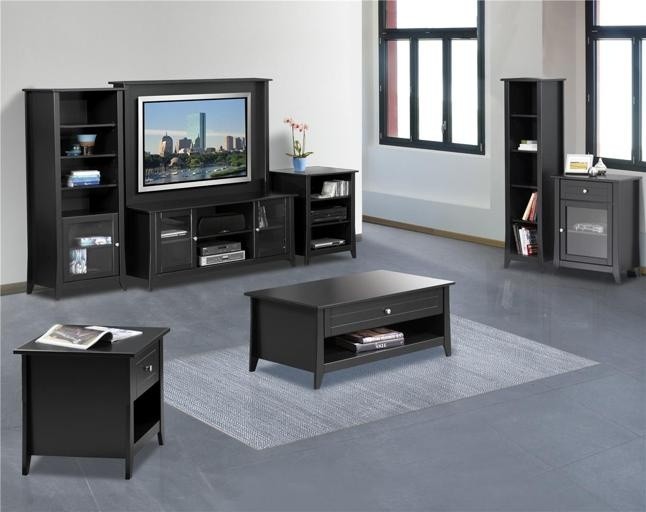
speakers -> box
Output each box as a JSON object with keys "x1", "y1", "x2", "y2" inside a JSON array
[{"x1": 197, "y1": 212, "x2": 246, "y2": 236}]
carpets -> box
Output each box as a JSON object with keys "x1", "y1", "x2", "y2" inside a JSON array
[{"x1": 156, "y1": 311, "x2": 602, "y2": 454}]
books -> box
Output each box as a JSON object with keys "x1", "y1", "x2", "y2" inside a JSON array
[
  {"x1": 35, "y1": 322, "x2": 144, "y2": 350},
  {"x1": 311, "y1": 178, "x2": 349, "y2": 199},
  {"x1": 330, "y1": 328, "x2": 405, "y2": 354},
  {"x1": 257, "y1": 201, "x2": 268, "y2": 230},
  {"x1": 61, "y1": 169, "x2": 101, "y2": 189},
  {"x1": 517, "y1": 139, "x2": 537, "y2": 152},
  {"x1": 513, "y1": 192, "x2": 539, "y2": 256},
  {"x1": 162, "y1": 228, "x2": 188, "y2": 239}
]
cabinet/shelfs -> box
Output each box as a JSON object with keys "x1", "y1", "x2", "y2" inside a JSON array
[
  {"x1": 498, "y1": 76, "x2": 566, "y2": 274},
  {"x1": 123, "y1": 190, "x2": 302, "y2": 292},
  {"x1": 548, "y1": 168, "x2": 643, "y2": 285},
  {"x1": 20, "y1": 85, "x2": 130, "y2": 301},
  {"x1": 10, "y1": 321, "x2": 167, "y2": 484},
  {"x1": 270, "y1": 163, "x2": 361, "y2": 267}
]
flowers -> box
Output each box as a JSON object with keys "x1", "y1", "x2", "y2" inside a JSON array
[{"x1": 281, "y1": 115, "x2": 317, "y2": 159}]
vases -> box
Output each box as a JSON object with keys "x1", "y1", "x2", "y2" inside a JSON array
[{"x1": 289, "y1": 157, "x2": 310, "y2": 172}]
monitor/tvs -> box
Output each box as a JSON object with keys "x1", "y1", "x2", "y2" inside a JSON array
[{"x1": 136, "y1": 92, "x2": 251, "y2": 194}]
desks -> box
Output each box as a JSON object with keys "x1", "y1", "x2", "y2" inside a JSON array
[{"x1": 240, "y1": 270, "x2": 458, "y2": 392}]
